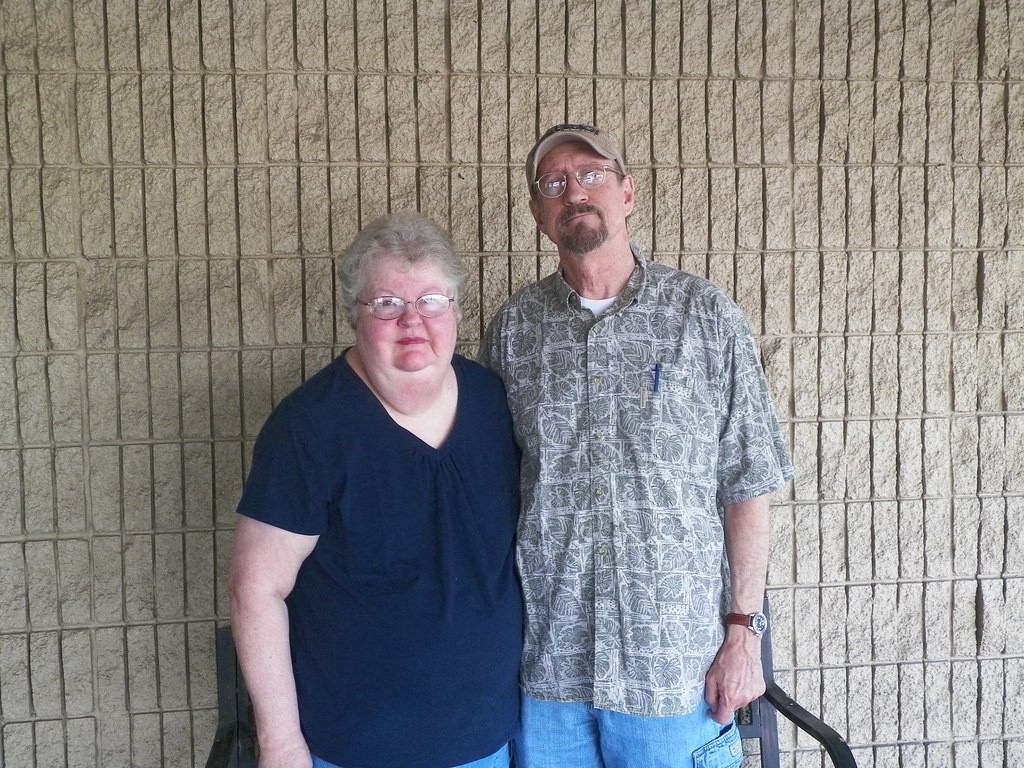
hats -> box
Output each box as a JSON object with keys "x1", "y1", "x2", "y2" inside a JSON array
[{"x1": 525, "y1": 123, "x2": 627, "y2": 197}]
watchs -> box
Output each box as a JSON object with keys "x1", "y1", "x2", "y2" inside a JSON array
[{"x1": 723, "y1": 611, "x2": 768, "y2": 637}]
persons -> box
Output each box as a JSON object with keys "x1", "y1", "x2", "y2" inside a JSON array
[
  {"x1": 473, "y1": 123, "x2": 799, "y2": 768},
  {"x1": 231, "y1": 210, "x2": 527, "y2": 768}
]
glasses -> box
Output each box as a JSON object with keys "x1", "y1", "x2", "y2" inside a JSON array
[
  {"x1": 534, "y1": 163, "x2": 626, "y2": 199},
  {"x1": 354, "y1": 293, "x2": 455, "y2": 320}
]
